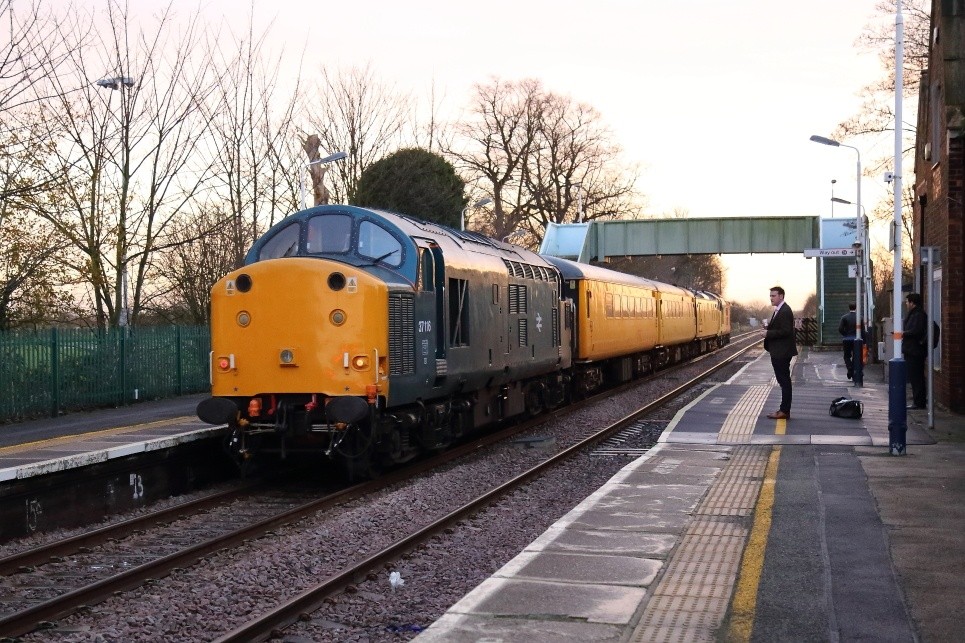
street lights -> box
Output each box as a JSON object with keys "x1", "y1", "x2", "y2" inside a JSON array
[
  {"x1": 299, "y1": 152, "x2": 348, "y2": 210},
  {"x1": 831, "y1": 197, "x2": 869, "y2": 365},
  {"x1": 843, "y1": 222, "x2": 873, "y2": 333},
  {"x1": 96, "y1": 76, "x2": 134, "y2": 405},
  {"x1": 808, "y1": 134, "x2": 864, "y2": 388}
]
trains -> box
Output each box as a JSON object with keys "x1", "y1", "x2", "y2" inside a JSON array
[{"x1": 195, "y1": 205, "x2": 732, "y2": 486}]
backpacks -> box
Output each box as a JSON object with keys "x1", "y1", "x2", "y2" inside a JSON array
[{"x1": 915, "y1": 315, "x2": 941, "y2": 350}]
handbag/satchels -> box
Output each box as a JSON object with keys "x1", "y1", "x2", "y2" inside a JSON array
[{"x1": 829, "y1": 397, "x2": 865, "y2": 419}]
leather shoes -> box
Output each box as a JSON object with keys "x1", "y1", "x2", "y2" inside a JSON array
[
  {"x1": 766, "y1": 409, "x2": 790, "y2": 419},
  {"x1": 906, "y1": 404, "x2": 926, "y2": 409},
  {"x1": 847, "y1": 367, "x2": 854, "y2": 379}
]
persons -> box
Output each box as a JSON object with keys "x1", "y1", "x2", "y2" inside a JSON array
[
  {"x1": 760, "y1": 287, "x2": 798, "y2": 418},
  {"x1": 839, "y1": 303, "x2": 866, "y2": 379},
  {"x1": 903, "y1": 292, "x2": 928, "y2": 409}
]
